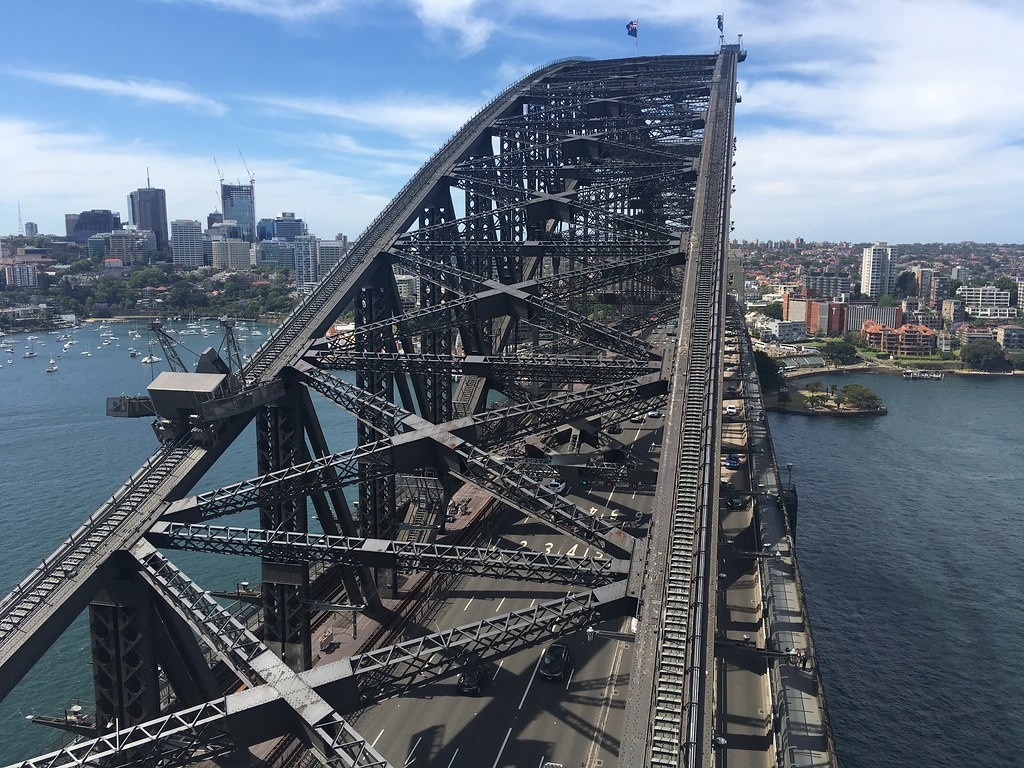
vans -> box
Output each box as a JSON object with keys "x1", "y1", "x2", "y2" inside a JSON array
[
  {"x1": 648, "y1": 404, "x2": 659, "y2": 417},
  {"x1": 724, "y1": 363, "x2": 735, "y2": 372},
  {"x1": 724, "y1": 450, "x2": 743, "y2": 470}
]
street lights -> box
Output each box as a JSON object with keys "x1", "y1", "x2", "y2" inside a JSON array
[{"x1": 787, "y1": 463, "x2": 795, "y2": 491}]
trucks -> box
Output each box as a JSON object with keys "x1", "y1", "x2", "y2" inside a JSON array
[{"x1": 665, "y1": 325, "x2": 676, "y2": 335}]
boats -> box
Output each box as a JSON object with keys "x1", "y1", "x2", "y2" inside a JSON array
[
  {"x1": 902, "y1": 370, "x2": 944, "y2": 380},
  {"x1": 0, "y1": 309, "x2": 273, "y2": 372}
]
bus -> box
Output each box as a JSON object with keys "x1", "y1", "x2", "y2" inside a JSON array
[{"x1": 785, "y1": 366, "x2": 798, "y2": 370}]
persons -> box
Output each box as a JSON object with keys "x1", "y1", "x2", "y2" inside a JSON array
[
  {"x1": 801, "y1": 648, "x2": 808, "y2": 671},
  {"x1": 784, "y1": 647, "x2": 790, "y2": 670},
  {"x1": 794, "y1": 648, "x2": 800, "y2": 670},
  {"x1": 777, "y1": 495, "x2": 780, "y2": 509}
]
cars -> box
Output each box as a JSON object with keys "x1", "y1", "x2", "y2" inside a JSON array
[
  {"x1": 652, "y1": 329, "x2": 658, "y2": 334},
  {"x1": 658, "y1": 325, "x2": 662, "y2": 329},
  {"x1": 608, "y1": 422, "x2": 623, "y2": 435},
  {"x1": 725, "y1": 496, "x2": 747, "y2": 510},
  {"x1": 629, "y1": 410, "x2": 642, "y2": 422},
  {"x1": 535, "y1": 640, "x2": 570, "y2": 680},
  {"x1": 726, "y1": 343, "x2": 735, "y2": 352}
]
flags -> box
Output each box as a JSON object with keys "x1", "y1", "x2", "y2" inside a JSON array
[
  {"x1": 626, "y1": 21, "x2": 637, "y2": 37},
  {"x1": 717, "y1": 15, "x2": 723, "y2": 32}
]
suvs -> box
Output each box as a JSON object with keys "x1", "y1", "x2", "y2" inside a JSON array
[
  {"x1": 726, "y1": 404, "x2": 737, "y2": 416},
  {"x1": 548, "y1": 475, "x2": 567, "y2": 494}
]
